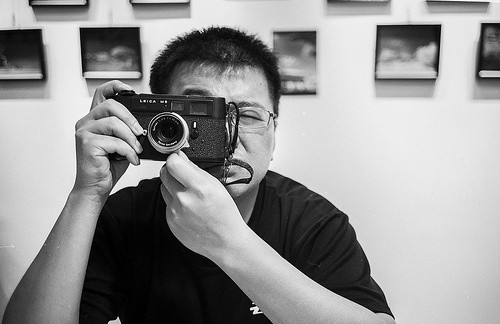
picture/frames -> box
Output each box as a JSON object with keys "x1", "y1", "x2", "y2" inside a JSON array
[
  {"x1": 475, "y1": 22, "x2": 500, "y2": 80},
  {"x1": 0, "y1": 29, "x2": 46, "y2": 82},
  {"x1": 80, "y1": 26, "x2": 143, "y2": 81},
  {"x1": 272, "y1": 31, "x2": 317, "y2": 96},
  {"x1": 374, "y1": 24, "x2": 442, "y2": 81}
]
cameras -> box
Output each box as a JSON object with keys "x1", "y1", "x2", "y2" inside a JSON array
[{"x1": 106, "y1": 90, "x2": 230, "y2": 165}]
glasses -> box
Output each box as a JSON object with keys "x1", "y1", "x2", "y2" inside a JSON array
[{"x1": 232, "y1": 107, "x2": 277, "y2": 129}]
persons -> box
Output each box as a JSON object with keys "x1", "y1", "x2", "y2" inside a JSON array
[{"x1": 2, "y1": 24, "x2": 398, "y2": 324}]
